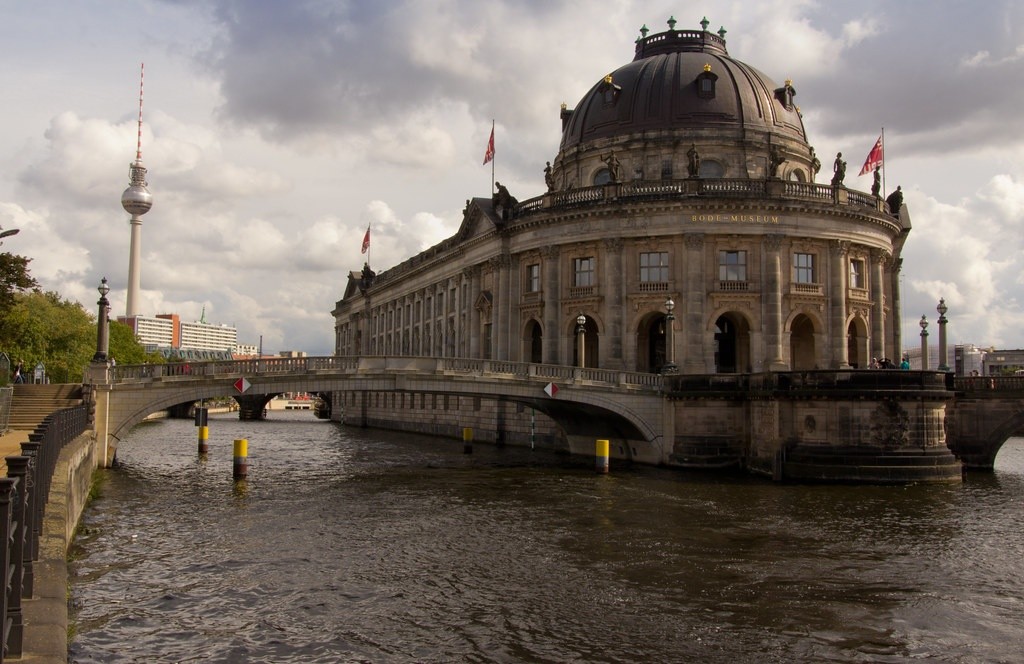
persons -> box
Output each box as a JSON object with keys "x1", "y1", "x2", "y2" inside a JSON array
[
  {"x1": 867, "y1": 357, "x2": 909, "y2": 370},
  {"x1": 686, "y1": 141, "x2": 701, "y2": 178},
  {"x1": 600, "y1": 148, "x2": 621, "y2": 183},
  {"x1": 544, "y1": 161, "x2": 556, "y2": 193},
  {"x1": 111, "y1": 357, "x2": 116, "y2": 367},
  {"x1": 12, "y1": 361, "x2": 24, "y2": 383},
  {"x1": 769, "y1": 144, "x2": 903, "y2": 213},
  {"x1": 494, "y1": 181, "x2": 511, "y2": 209},
  {"x1": 143, "y1": 360, "x2": 148, "y2": 374}
]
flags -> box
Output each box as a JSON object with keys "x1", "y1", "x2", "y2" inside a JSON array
[
  {"x1": 361, "y1": 227, "x2": 371, "y2": 254},
  {"x1": 857, "y1": 135, "x2": 883, "y2": 178},
  {"x1": 482, "y1": 127, "x2": 495, "y2": 165}
]
